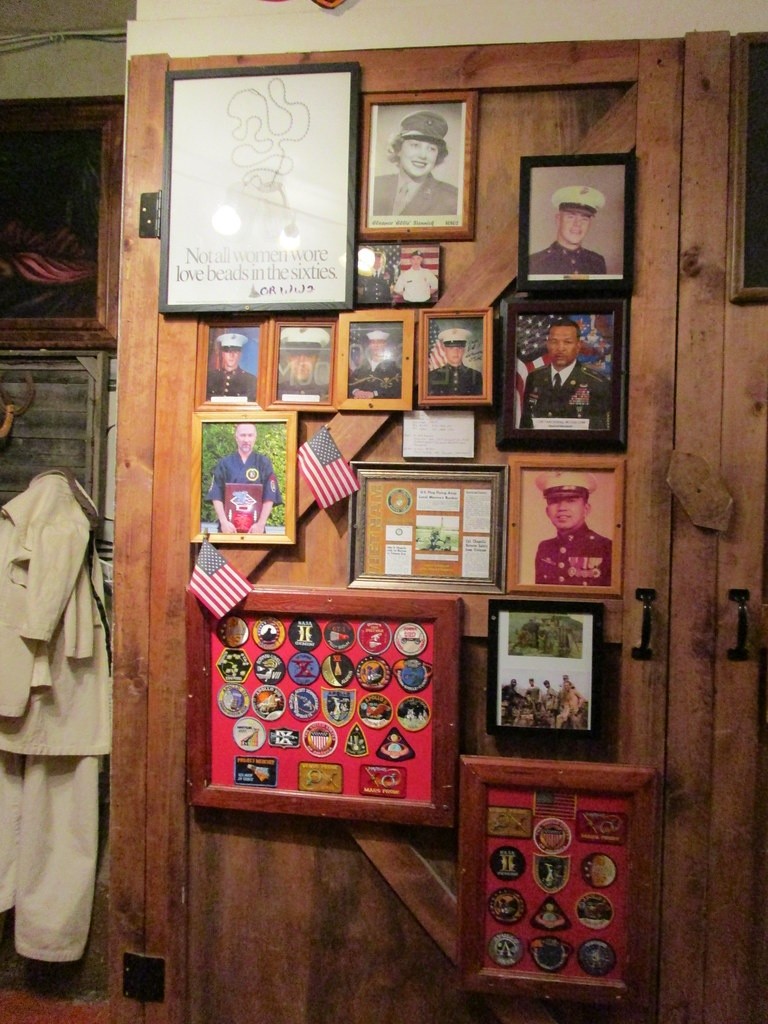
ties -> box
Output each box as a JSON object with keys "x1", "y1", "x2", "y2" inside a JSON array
[
  {"x1": 375, "y1": 271, "x2": 378, "y2": 278},
  {"x1": 553, "y1": 373, "x2": 562, "y2": 397}
]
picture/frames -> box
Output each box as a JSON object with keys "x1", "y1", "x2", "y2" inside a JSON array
[
  {"x1": 506, "y1": 453, "x2": 626, "y2": 595},
  {"x1": 158, "y1": 60, "x2": 361, "y2": 313},
  {"x1": 188, "y1": 592, "x2": 460, "y2": 828},
  {"x1": 730, "y1": 29, "x2": 768, "y2": 309},
  {"x1": 266, "y1": 316, "x2": 339, "y2": 413},
  {"x1": 518, "y1": 151, "x2": 637, "y2": 292},
  {"x1": 356, "y1": 92, "x2": 478, "y2": 242},
  {"x1": 189, "y1": 412, "x2": 297, "y2": 546},
  {"x1": 485, "y1": 601, "x2": 605, "y2": 737},
  {"x1": 354, "y1": 469, "x2": 501, "y2": 586},
  {"x1": 195, "y1": 318, "x2": 268, "y2": 412},
  {"x1": 455, "y1": 760, "x2": 659, "y2": 998},
  {"x1": 0, "y1": 94, "x2": 122, "y2": 349},
  {"x1": 338, "y1": 310, "x2": 414, "y2": 412},
  {"x1": 497, "y1": 298, "x2": 632, "y2": 449},
  {"x1": 417, "y1": 307, "x2": 494, "y2": 405}
]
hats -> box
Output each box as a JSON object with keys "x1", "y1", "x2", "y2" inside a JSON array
[
  {"x1": 280, "y1": 326, "x2": 331, "y2": 355},
  {"x1": 399, "y1": 110, "x2": 448, "y2": 147},
  {"x1": 411, "y1": 249, "x2": 424, "y2": 257},
  {"x1": 217, "y1": 332, "x2": 248, "y2": 351},
  {"x1": 438, "y1": 328, "x2": 473, "y2": 347},
  {"x1": 536, "y1": 467, "x2": 598, "y2": 498},
  {"x1": 374, "y1": 247, "x2": 383, "y2": 256},
  {"x1": 551, "y1": 184, "x2": 606, "y2": 217},
  {"x1": 366, "y1": 329, "x2": 390, "y2": 341}
]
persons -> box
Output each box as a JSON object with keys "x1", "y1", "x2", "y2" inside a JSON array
[
  {"x1": 427, "y1": 328, "x2": 483, "y2": 396},
  {"x1": 394, "y1": 250, "x2": 439, "y2": 302},
  {"x1": 519, "y1": 319, "x2": 610, "y2": 430},
  {"x1": 533, "y1": 469, "x2": 611, "y2": 586},
  {"x1": 206, "y1": 334, "x2": 258, "y2": 403},
  {"x1": 373, "y1": 110, "x2": 457, "y2": 216},
  {"x1": 501, "y1": 674, "x2": 588, "y2": 729},
  {"x1": 348, "y1": 331, "x2": 400, "y2": 398},
  {"x1": 204, "y1": 422, "x2": 282, "y2": 534},
  {"x1": 277, "y1": 328, "x2": 330, "y2": 399},
  {"x1": 520, "y1": 615, "x2": 563, "y2": 659},
  {"x1": 527, "y1": 185, "x2": 607, "y2": 273},
  {"x1": 356, "y1": 248, "x2": 393, "y2": 302}
]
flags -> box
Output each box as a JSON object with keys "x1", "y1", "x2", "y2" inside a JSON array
[
  {"x1": 349, "y1": 322, "x2": 369, "y2": 376},
  {"x1": 517, "y1": 315, "x2": 562, "y2": 429},
  {"x1": 295, "y1": 426, "x2": 360, "y2": 510},
  {"x1": 428, "y1": 319, "x2": 447, "y2": 370},
  {"x1": 186, "y1": 538, "x2": 256, "y2": 621},
  {"x1": 357, "y1": 245, "x2": 439, "y2": 302}
]
